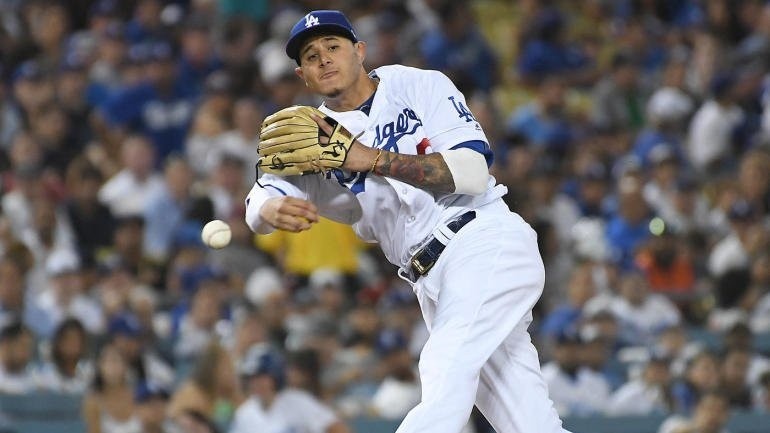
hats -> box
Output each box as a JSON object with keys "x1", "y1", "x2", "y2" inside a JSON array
[{"x1": 286, "y1": 10, "x2": 357, "y2": 60}]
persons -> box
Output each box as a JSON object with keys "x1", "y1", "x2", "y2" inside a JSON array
[
  {"x1": 244, "y1": 11, "x2": 572, "y2": 433},
  {"x1": 0, "y1": 0, "x2": 770, "y2": 433}
]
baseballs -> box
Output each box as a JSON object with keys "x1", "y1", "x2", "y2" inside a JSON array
[{"x1": 202, "y1": 220, "x2": 232, "y2": 250}]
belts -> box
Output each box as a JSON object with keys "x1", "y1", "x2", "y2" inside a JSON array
[{"x1": 411, "y1": 211, "x2": 475, "y2": 282}]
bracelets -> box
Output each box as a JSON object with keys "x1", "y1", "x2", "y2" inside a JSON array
[{"x1": 370, "y1": 147, "x2": 382, "y2": 174}]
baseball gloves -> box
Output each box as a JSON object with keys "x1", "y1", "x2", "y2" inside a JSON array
[{"x1": 257, "y1": 105, "x2": 355, "y2": 176}]
